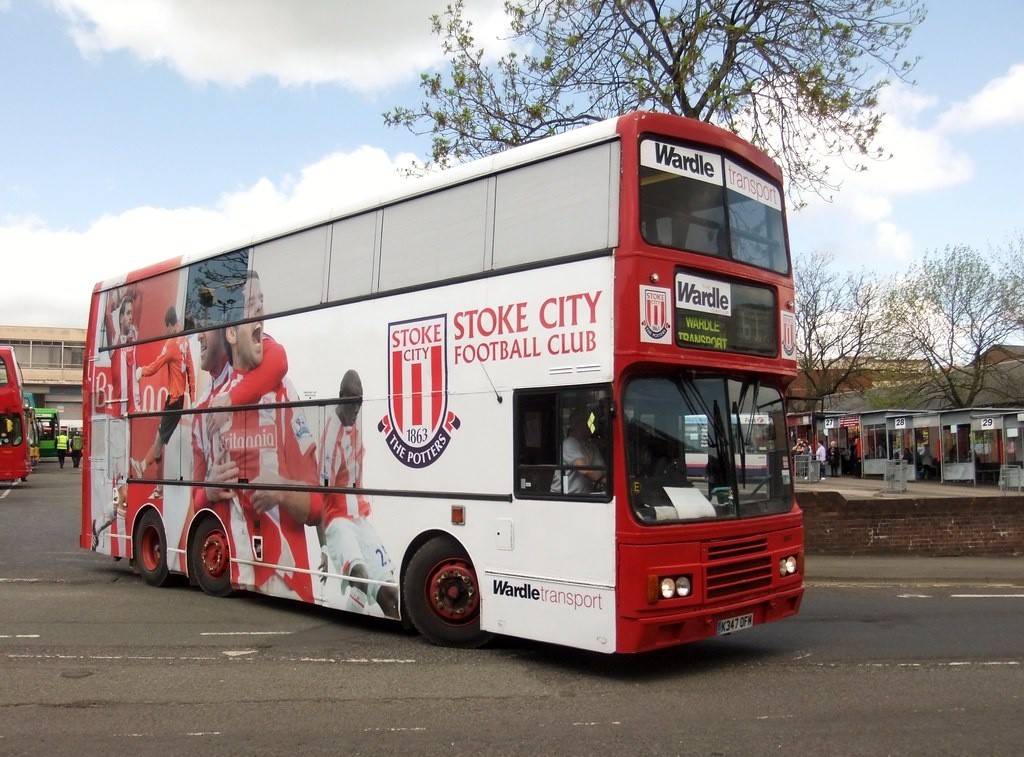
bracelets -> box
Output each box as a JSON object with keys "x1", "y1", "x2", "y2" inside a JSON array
[
  {"x1": 321, "y1": 545, "x2": 329, "y2": 557},
  {"x1": 193, "y1": 486, "x2": 214, "y2": 514}
]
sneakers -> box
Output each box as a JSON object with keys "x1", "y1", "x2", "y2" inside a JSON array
[
  {"x1": 130, "y1": 457, "x2": 143, "y2": 479},
  {"x1": 149, "y1": 488, "x2": 164, "y2": 499},
  {"x1": 92, "y1": 519, "x2": 99, "y2": 550}
]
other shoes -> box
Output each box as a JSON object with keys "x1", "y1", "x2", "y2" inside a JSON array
[
  {"x1": 821, "y1": 476, "x2": 826, "y2": 479},
  {"x1": 804, "y1": 475, "x2": 808, "y2": 479}
]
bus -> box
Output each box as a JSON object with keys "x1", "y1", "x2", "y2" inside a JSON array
[
  {"x1": 0, "y1": 345, "x2": 82, "y2": 482},
  {"x1": 81, "y1": 110, "x2": 807, "y2": 656},
  {"x1": 684, "y1": 413, "x2": 775, "y2": 483}
]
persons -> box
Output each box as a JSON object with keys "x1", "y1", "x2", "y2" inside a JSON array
[
  {"x1": 11, "y1": 476, "x2": 27, "y2": 482},
  {"x1": 71, "y1": 431, "x2": 82, "y2": 468},
  {"x1": 92, "y1": 270, "x2": 400, "y2": 619},
  {"x1": 550, "y1": 399, "x2": 674, "y2": 494},
  {"x1": 791, "y1": 436, "x2": 981, "y2": 480},
  {"x1": 54, "y1": 431, "x2": 69, "y2": 469}
]
